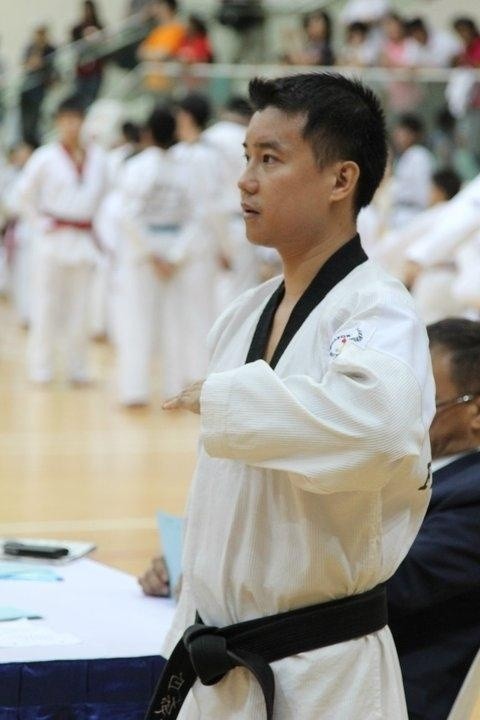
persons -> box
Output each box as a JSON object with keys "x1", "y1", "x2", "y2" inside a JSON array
[
  {"x1": 146, "y1": 75, "x2": 436, "y2": 718},
  {"x1": 140, "y1": 314, "x2": 480, "y2": 720},
  {"x1": 0, "y1": 1, "x2": 480, "y2": 408}
]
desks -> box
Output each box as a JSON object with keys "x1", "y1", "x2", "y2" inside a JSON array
[{"x1": 0, "y1": 543, "x2": 184, "y2": 720}]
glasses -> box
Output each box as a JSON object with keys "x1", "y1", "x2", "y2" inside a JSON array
[{"x1": 434, "y1": 392, "x2": 472, "y2": 417}]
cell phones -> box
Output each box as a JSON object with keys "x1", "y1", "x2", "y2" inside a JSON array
[{"x1": 3, "y1": 542, "x2": 68, "y2": 559}]
